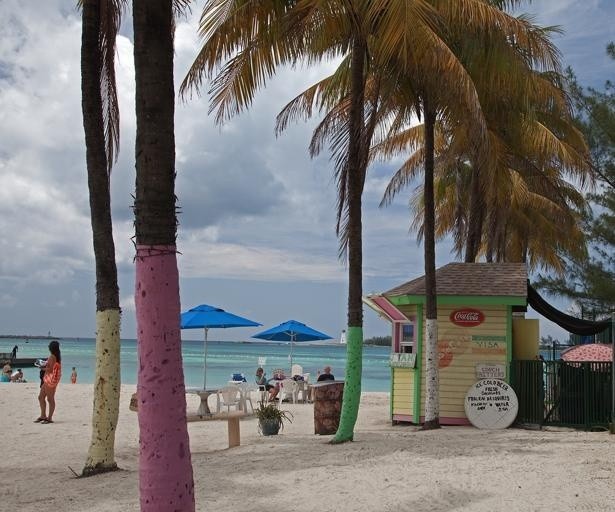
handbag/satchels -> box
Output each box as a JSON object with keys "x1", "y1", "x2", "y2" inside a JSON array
[{"x1": 41, "y1": 361, "x2": 62, "y2": 389}]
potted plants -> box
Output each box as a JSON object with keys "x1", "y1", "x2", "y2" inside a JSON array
[{"x1": 252, "y1": 392, "x2": 294, "y2": 437}]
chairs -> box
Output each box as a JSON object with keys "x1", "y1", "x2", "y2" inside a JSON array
[{"x1": 216, "y1": 363, "x2": 316, "y2": 414}]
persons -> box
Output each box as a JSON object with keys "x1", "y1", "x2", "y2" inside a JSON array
[
  {"x1": 255, "y1": 368, "x2": 279, "y2": 402},
  {"x1": 317, "y1": 367, "x2": 334, "y2": 381},
  {"x1": 0, "y1": 345, "x2": 23, "y2": 382},
  {"x1": 71, "y1": 367, "x2": 77, "y2": 384},
  {"x1": 33, "y1": 341, "x2": 61, "y2": 424}
]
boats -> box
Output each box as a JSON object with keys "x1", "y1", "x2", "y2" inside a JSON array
[{"x1": 0, "y1": 351, "x2": 48, "y2": 367}]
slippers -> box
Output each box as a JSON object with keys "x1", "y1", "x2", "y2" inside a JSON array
[{"x1": 34, "y1": 418, "x2": 55, "y2": 426}]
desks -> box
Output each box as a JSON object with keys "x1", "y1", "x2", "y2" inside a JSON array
[{"x1": 186, "y1": 388, "x2": 222, "y2": 415}]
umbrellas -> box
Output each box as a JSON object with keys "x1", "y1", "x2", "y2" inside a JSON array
[
  {"x1": 251, "y1": 319, "x2": 335, "y2": 373},
  {"x1": 180, "y1": 304, "x2": 264, "y2": 390}
]
